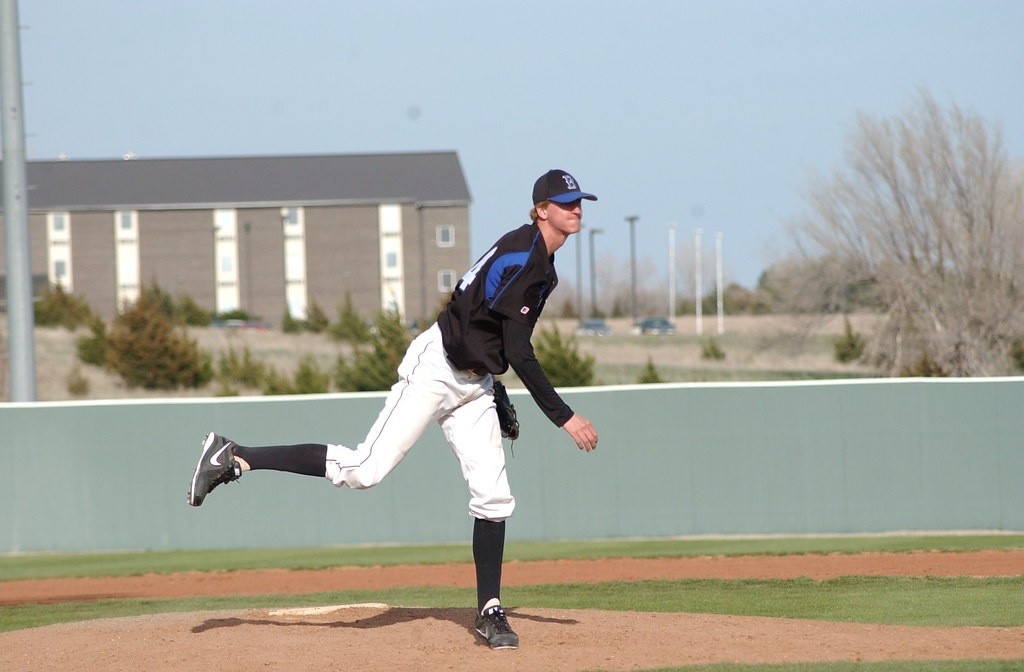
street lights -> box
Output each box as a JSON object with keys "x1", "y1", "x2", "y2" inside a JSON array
[
  {"x1": 589, "y1": 229, "x2": 604, "y2": 314},
  {"x1": 712, "y1": 233, "x2": 724, "y2": 336},
  {"x1": 626, "y1": 214, "x2": 640, "y2": 320},
  {"x1": 667, "y1": 224, "x2": 676, "y2": 316},
  {"x1": 693, "y1": 226, "x2": 703, "y2": 335}
]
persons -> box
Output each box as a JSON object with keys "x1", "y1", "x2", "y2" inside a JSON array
[{"x1": 186, "y1": 168, "x2": 599, "y2": 651}]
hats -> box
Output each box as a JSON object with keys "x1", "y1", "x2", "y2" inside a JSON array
[{"x1": 532, "y1": 169, "x2": 599, "y2": 205}]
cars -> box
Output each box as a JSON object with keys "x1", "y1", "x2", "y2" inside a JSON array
[
  {"x1": 575, "y1": 321, "x2": 612, "y2": 337},
  {"x1": 632, "y1": 315, "x2": 676, "y2": 337}
]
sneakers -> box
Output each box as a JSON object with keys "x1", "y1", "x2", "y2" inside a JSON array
[
  {"x1": 187, "y1": 432, "x2": 242, "y2": 506},
  {"x1": 473, "y1": 604, "x2": 519, "y2": 649}
]
